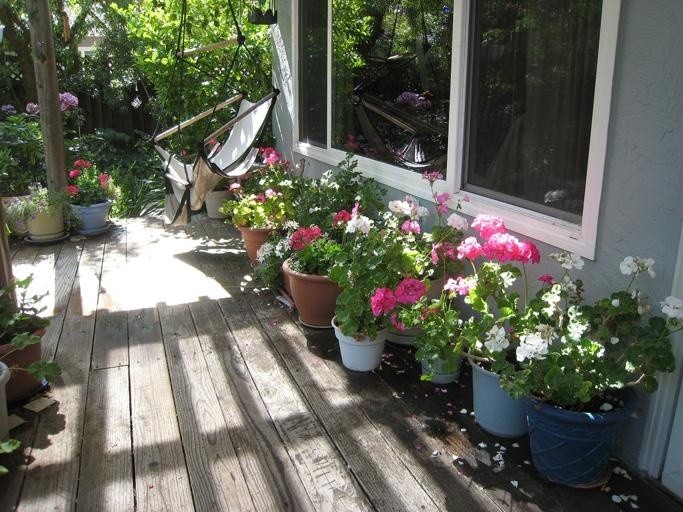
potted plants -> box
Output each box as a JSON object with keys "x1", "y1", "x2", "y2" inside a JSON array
[
  {"x1": 0, "y1": 326, "x2": 62, "y2": 480},
  {"x1": 0, "y1": 266, "x2": 65, "y2": 403}
]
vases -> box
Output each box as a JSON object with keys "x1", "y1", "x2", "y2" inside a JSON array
[
  {"x1": 205, "y1": 192, "x2": 231, "y2": 219},
  {"x1": 468, "y1": 348, "x2": 533, "y2": 439},
  {"x1": 332, "y1": 314, "x2": 388, "y2": 371},
  {"x1": 524, "y1": 398, "x2": 634, "y2": 487}
]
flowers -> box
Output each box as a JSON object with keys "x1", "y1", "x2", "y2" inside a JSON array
[
  {"x1": 229, "y1": 146, "x2": 470, "y2": 342},
  {"x1": 367, "y1": 170, "x2": 683, "y2": 402},
  {"x1": 179, "y1": 138, "x2": 225, "y2": 190},
  {"x1": 0, "y1": 90, "x2": 120, "y2": 245}
]
